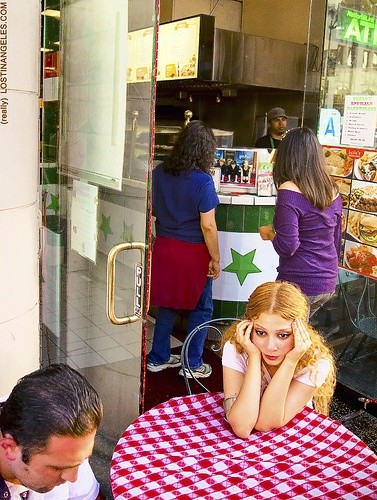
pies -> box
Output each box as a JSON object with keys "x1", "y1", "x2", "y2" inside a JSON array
[{"x1": 349, "y1": 185, "x2": 377, "y2": 213}]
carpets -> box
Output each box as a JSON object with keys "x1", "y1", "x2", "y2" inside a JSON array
[{"x1": 76, "y1": 344, "x2": 223, "y2": 439}]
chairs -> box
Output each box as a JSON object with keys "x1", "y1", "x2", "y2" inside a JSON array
[
  {"x1": 335, "y1": 268, "x2": 377, "y2": 368},
  {"x1": 181, "y1": 317, "x2": 243, "y2": 396}
]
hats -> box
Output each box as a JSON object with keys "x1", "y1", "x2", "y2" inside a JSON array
[{"x1": 267, "y1": 107, "x2": 288, "y2": 122}]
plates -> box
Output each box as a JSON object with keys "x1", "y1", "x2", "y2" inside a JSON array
[
  {"x1": 347, "y1": 180, "x2": 377, "y2": 214},
  {"x1": 346, "y1": 212, "x2": 376, "y2": 249},
  {"x1": 344, "y1": 240, "x2": 377, "y2": 279},
  {"x1": 354, "y1": 152, "x2": 377, "y2": 182},
  {"x1": 323, "y1": 149, "x2": 353, "y2": 177}
]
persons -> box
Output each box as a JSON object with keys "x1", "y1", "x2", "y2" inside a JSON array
[
  {"x1": 147, "y1": 120, "x2": 221, "y2": 377},
  {"x1": 214, "y1": 158, "x2": 251, "y2": 185},
  {"x1": 0, "y1": 363, "x2": 102, "y2": 500},
  {"x1": 255, "y1": 108, "x2": 288, "y2": 148},
  {"x1": 222, "y1": 282, "x2": 337, "y2": 439},
  {"x1": 260, "y1": 127, "x2": 342, "y2": 319}
]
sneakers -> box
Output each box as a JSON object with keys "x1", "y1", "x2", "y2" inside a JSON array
[
  {"x1": 179, "y1": 363, "x2": 212, "y2": 378},
  {"x1": 147, "y1": 353, "x2": 182, "y2": 372}
]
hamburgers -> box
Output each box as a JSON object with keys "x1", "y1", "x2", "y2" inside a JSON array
[{"x1": 359, "y1": 217, "x2": 377, "y2": 246}]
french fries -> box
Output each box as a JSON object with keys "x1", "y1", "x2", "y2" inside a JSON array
[{"x1": 349, "y1": 211, "x2": 363, "y2": 237}]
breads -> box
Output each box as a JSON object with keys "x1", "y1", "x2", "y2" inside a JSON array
[{"x1": 320, "y1": 146, "x2": 347, "y2": 168}]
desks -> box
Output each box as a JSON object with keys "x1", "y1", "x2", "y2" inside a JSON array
[{"x1": 109, "y1": 391, "x2": 377, "y2": 500}]
